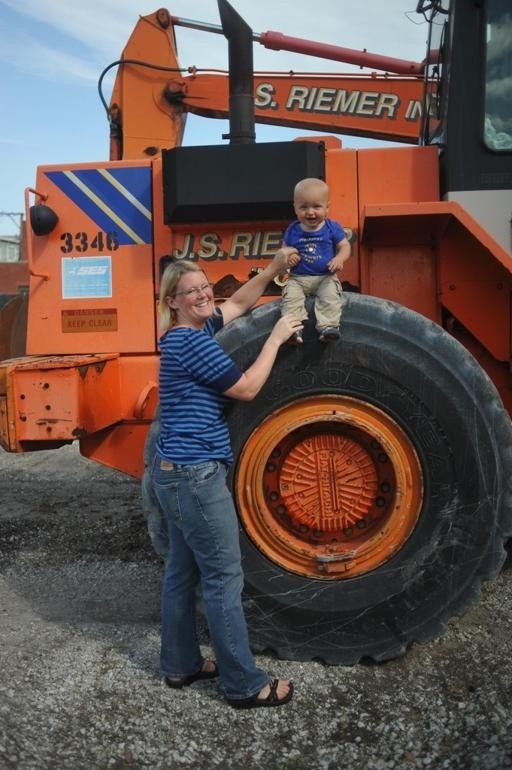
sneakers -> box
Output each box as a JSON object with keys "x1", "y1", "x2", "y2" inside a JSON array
[
  {"x1": 318, "y1": 327, "x2": 340, "y2": 342},
  {"x1": 288, "y1": 330, "x2": 303, "y2": 345}
]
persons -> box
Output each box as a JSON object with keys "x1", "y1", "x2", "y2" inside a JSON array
[
  {"x1": 270, "y1": 177, "x2": 353, "y2": 346},
  {"x1": 147, "y1": 244, "x2": 304, "y2": 711}
]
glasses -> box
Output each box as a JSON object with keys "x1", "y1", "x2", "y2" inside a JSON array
[{"x1": 174, "y1": 282, "x2": 213, "y2": 295}]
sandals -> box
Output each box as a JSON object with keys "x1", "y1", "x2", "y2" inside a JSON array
[
  {"x1": 166, "y1": 657, "x2": 218, "y2": 688},
  {"x1": 228, "y1": 678, "x2": 293, "y2": 710}
]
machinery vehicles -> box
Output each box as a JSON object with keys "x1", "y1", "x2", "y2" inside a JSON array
[{"x1": 0, "y1": 0, "x2": 512, "y2": 668}]
